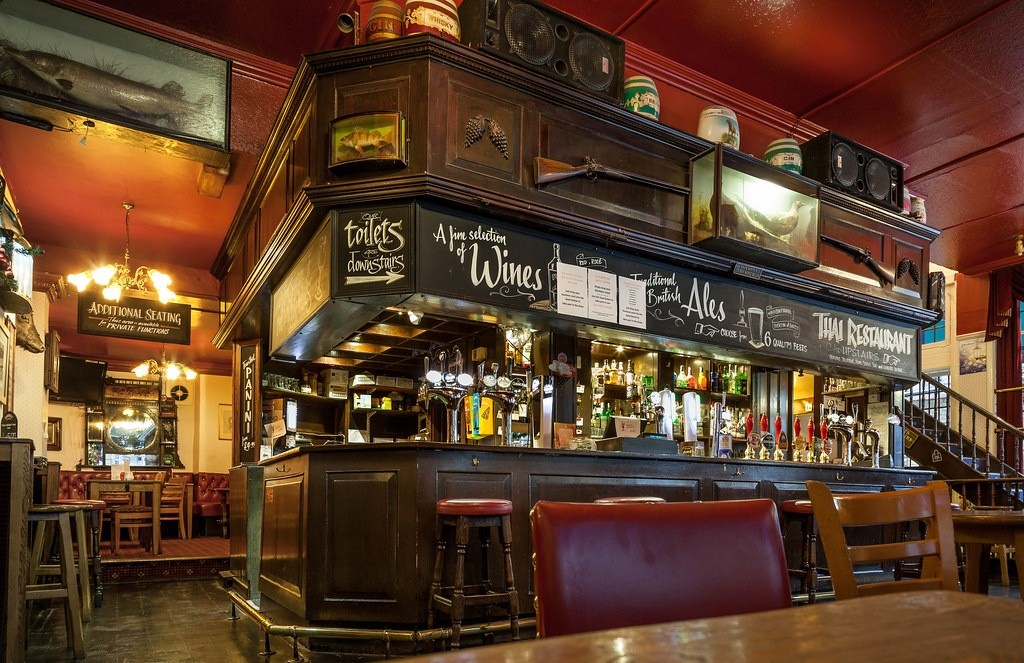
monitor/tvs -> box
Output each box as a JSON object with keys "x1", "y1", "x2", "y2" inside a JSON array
[{"x1": 48, "y1": 355, "x2": 108, "y2": 406}]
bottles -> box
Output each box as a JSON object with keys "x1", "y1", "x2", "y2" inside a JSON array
[
  {"x1": 262, "y1": 372, "x2": 312, "y2": 394},
  {"x1": 590, "y1": 359, "x2": 654, "y2": 429},
  {"x1": 824, "y1": 377, "x2": 865, "y2": 392},
  {"x1": 672, "y1": 364, "x2": 747, "y2": 438}
]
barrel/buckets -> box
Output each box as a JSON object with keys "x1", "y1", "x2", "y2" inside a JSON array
[
  {"x1": 901, "y1": 184, "x2": 927, "y2": 224},
  {"x1": 624, "y1": 76, "x2": 660, "y2": 120},
  {"x1": 697, "y1": 105, "x2": 740, "y2": 150},
  {"x1": 764, "y1": 138, "x2": 802, "y2": 176},
  {"x1": 366, "y1": 0, "x2": 461, "y2": 44}
]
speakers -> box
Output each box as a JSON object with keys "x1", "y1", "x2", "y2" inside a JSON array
[
  {"x1": 800, "y1": 130, "x2": 904, "y2": 213},
  {"x1": 456, "y1": 0, "x2": 625, "y2": 108}
]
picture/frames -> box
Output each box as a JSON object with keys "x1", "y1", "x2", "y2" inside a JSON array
[
  {"x1": 0, "y1": 0, "x2": 232, "y2": 154},
  {"x1": 46, "y1": 417, "x2": 63, "y2": 451}
]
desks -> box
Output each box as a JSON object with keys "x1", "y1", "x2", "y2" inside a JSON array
[
  {"x1": 920, "y1": 510, "x2": 1024, "y2": 600},
  {"x1": 394, "y1": 591, "x2": 1024, "y2": 663},
  {"x1": 85, "y1": 478, "x2": 162, "y2": 556}
]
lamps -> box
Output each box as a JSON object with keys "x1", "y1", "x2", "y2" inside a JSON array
[
  {"x1": 134, "y1": 343, "x2": 198, "y2": 380},
  {"x1": 69, "y1": 203, "x2": 176, "y2": 302}
]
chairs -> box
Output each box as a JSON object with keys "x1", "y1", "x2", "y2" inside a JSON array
[
  {"x1": 58, "y1": 471, "x2": 229, "y2": 555},
  {"x1": 530, "y1": 498, "x2": 793, "y2": 635},
  {"x1": 805, "y1": 478, "x2": 961, "y2": 600}
]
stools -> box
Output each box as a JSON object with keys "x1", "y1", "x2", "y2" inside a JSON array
[
  {"x1": 428, "y1": 497, "x2": 521, "y2": 651},
  {"x1": 25, "y1": 500, "x2": 106, "y2": 658},
  {"x1": 782, "y1": 496, "x2": 831, "y2": 604}
]
